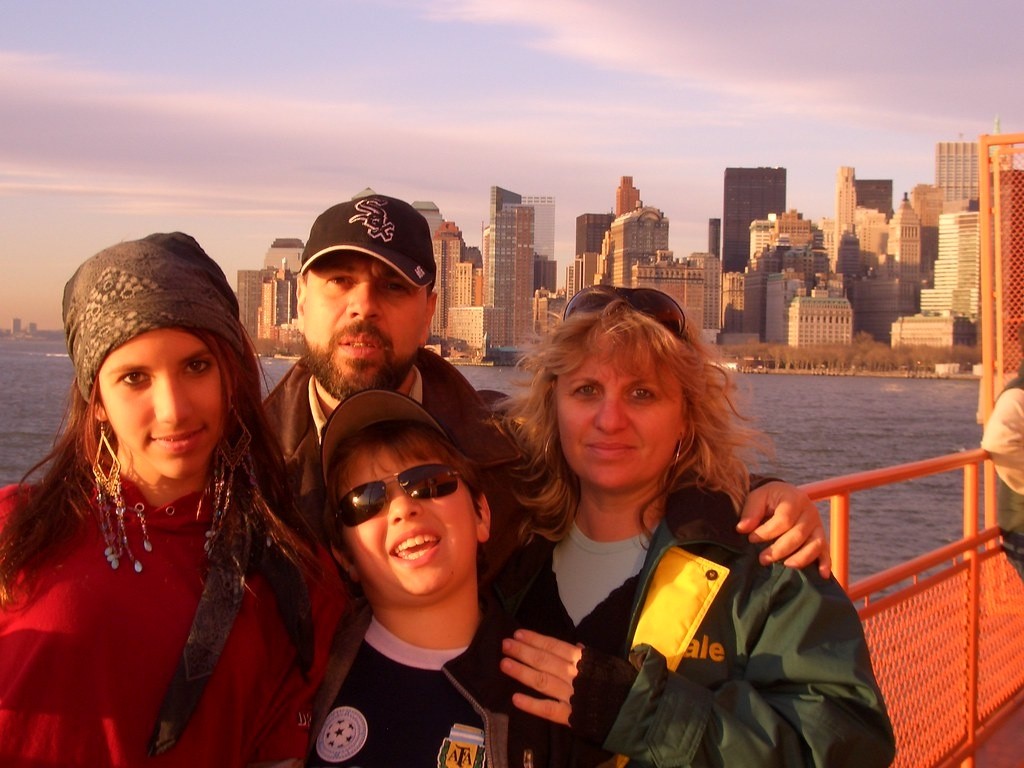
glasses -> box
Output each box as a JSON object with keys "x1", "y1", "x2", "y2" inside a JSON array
[
  {"x1": 332, "y1": 464, "x2": 462, "y2": 527},
  {"x1": 562, "y1": 283, "x2": 686, "y2": 336}
]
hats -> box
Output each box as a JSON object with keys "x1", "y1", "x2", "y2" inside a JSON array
[
  {"x1": 300, "y1": 194, "x2": 436, "y2": 287},
  {"x1": 319, "y1": 387, "x2": 452, "y2": 486}
]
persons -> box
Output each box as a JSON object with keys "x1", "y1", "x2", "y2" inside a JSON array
[
  {"x1": 0, "y1": 233, "x2": 350, "y2": 768},
  {"x1": 480, "y1": 285, "x2": 896, "y2": 768},
  {"x1": 262, "y1": 195, "x2": 832, "y2": 580},
  {"x1": 980, "y1": 322, "x2": 1024, "y2": 581},
  {"x1": 305, "y1": 385, "x2": 615, "y2": 768}
]
